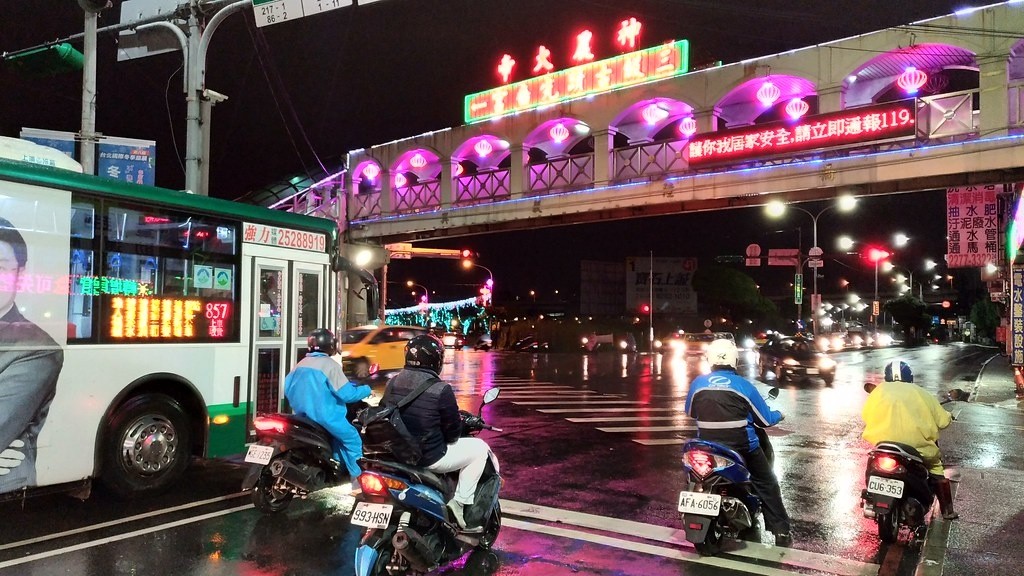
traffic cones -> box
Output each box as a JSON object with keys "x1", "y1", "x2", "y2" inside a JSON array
[{"x1": 1013, "y1": 366, "x2": 1024, "y2": 400}]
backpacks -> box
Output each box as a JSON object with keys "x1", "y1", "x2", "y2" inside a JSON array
[{"x1": 352, "y1": 377, "x2": 442, "y2": 466}]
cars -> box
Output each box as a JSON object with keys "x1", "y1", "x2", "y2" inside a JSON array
[
  {"x1": 429, "y1": 328, "x2": 457, "y2": 348},
  {"x1": 342, "y1": 324, "x2": 429, "y2": 384},
  {"x1": 659, "y1": 322, "x2": 950, "y2": 370},
  {"x1": 759, "y1": 335, "x2": 837, "y2": 389}
]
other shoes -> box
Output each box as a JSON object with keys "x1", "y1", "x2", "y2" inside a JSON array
[
  {"x1": 943, "y1": 510, "x2": 959, "y2": 519},
  {"x1": 775, "y1": 532, "x2": 793, "y2": 546},
  {"x1": 446, "y1": 499, "x2": 466, "y2": 529},
  {"x1": 350, "y1": 488, "x2": 363, "y2": 496}
]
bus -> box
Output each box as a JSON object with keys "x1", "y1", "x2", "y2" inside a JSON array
[{"x1": 0, "y1": 135, "x2": 379, "y2": 505}]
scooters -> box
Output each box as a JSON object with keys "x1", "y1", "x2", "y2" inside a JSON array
[
  {"x1": 860, "y1": 382, "x2": 965, "y2": 545},
  {"x1": 514, "y1": 336, "x2": 550, "y2": 354},
  {"x1": 678, "y1": 387, "x2": 780, "y2": 557},
  {"x1": 348, "y1": 385, "x2": 505, "y2": 576},
  {"x1": 579, "y1": 334, "x2": 639, "y2": 355},
  {"x1": 473, "y1": 336, "x2": 493, "y2": 352},
  {"x1": 453, "y1": 334, "x2": 464, "y2": 350},
  {"x1": 240, "y1": 363, "x2": 381, "y2": 515}
]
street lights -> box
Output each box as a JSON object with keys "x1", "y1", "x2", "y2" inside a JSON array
[
  {"x1": 464, "y1": 260, "x2": 493, "y2": 333},
  {"x1": 408, "y1": 281, "x2": 428, "y2": 302},
  {"x1": 766, "y1": 193, "x2": 855, "y2": 334},
  {"x1": 840, "y1": 232, "x2": 907, "y2": 330},
  {"x1": 882, "y1": 257, "x2": 935, "y2": 296}
]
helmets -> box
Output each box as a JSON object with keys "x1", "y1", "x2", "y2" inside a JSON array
[
  {"x1": 706, "y1": 338, "x2": 738, "y2": 371},
  {"x1": 885, "y1": 360, "x2": 913, "y2": 383},
  {"x1": 307, "y1": 329, "x2": 337, "y2": 357},
  {"x1": 405, "y1": 334, "x2": 445, "y2": 375}
]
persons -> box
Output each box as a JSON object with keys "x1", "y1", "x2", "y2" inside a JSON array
[
  {"x1": 861, "y1": 360, "x2": 959, "y2": 519},
  {"x1": 377, "y1": 335, "x2": 489, "y2": 528},
  {"x1": 0, "y1": 217, "x2": 65, "y2": 492},
  {"x1": 684, "y1": 339, "x2": 792, "y2": 546},
  {"x1": 282, "y1": 328, "x2": 372, "y2": 481}
]
